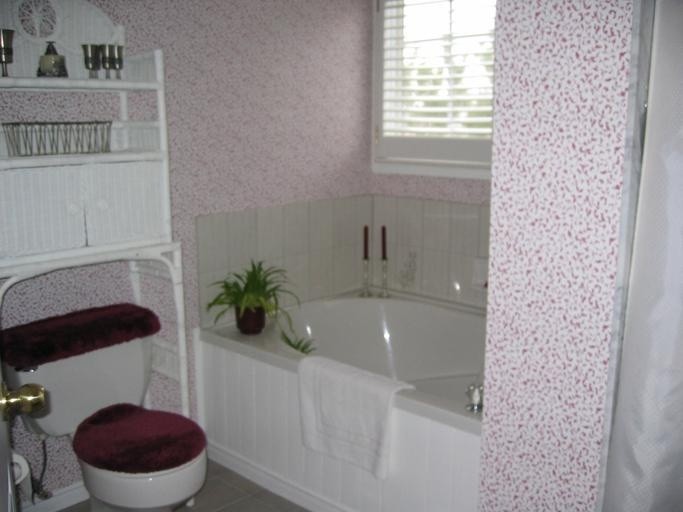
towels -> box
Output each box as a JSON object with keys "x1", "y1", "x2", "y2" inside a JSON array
[{"x1": 298, "y1": 354, "x2": 417, "y2": 479}]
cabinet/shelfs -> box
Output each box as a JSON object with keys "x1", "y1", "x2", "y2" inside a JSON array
[{"x1": 0, "y1": 1, "x2": 175, "y2": 283}]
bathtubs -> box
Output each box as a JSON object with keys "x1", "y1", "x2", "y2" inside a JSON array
[{"x1": 197, "y1": 284, "x2": 486, "y2": 436}]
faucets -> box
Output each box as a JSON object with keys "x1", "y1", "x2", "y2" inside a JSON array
[
  {"x1": 357, "y1": 225, "x2": 373, "y2": 298},
  {"x1": 378, "y1": 224, "x2": 390, "y2": 297}
]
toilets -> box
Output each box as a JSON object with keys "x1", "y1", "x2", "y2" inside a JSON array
[{"x1": 1, "y1": 302, "x2": 208, "y2": 512}]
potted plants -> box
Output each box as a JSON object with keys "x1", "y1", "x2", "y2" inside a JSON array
[{"x1": 204, "y1": 259, "x2": 296, "y2": 335}]
